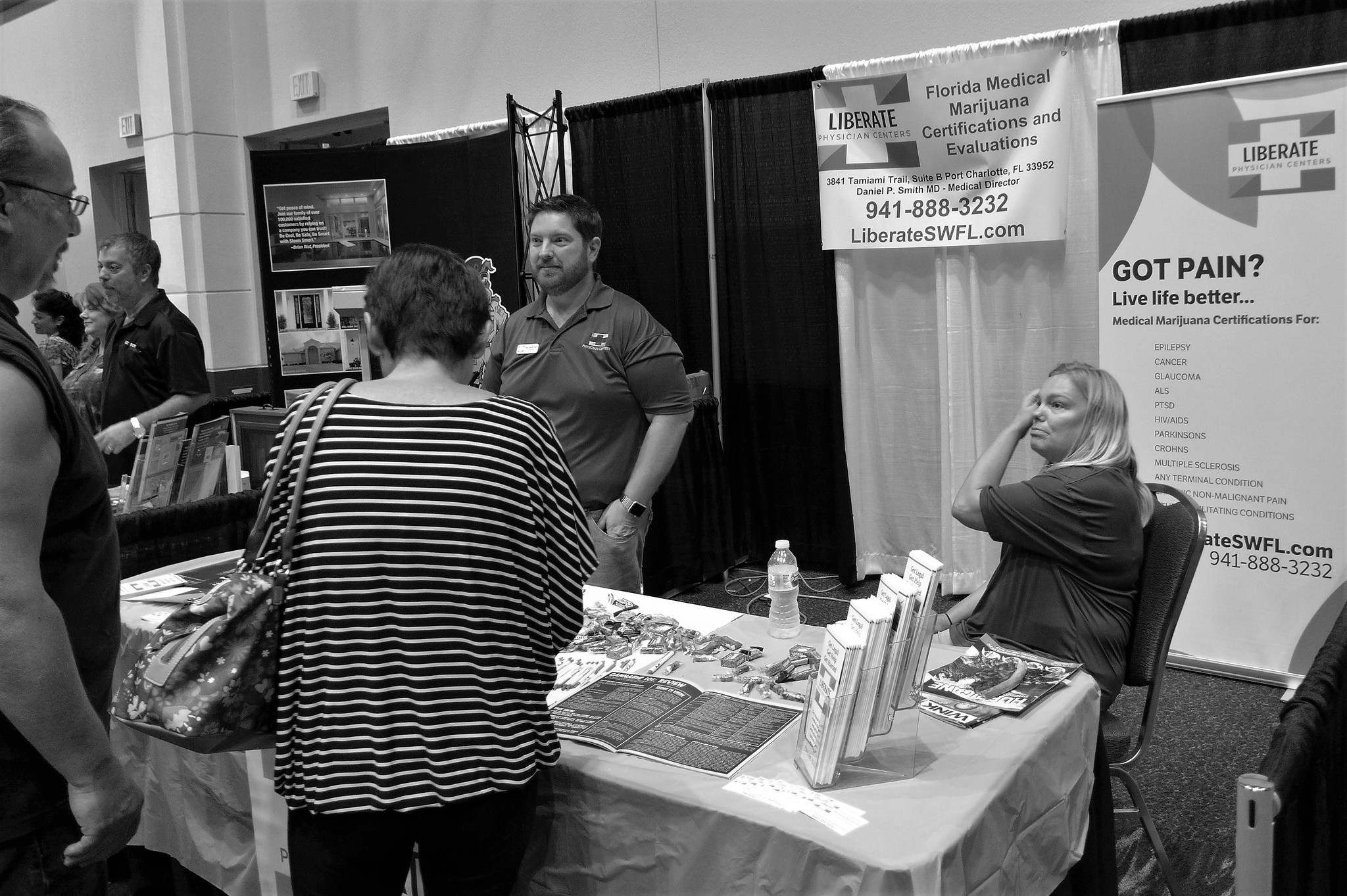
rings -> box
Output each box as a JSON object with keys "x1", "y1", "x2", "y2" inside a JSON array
[{"x1": 108, "y1": 445, "x2": 113, "y2": 451}]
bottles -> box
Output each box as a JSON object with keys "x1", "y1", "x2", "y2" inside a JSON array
[{"x1": 767, "y1": 540, "x2": 800, "y2": 639}]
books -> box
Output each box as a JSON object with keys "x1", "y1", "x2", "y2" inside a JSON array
[
  {"x1": 131, "y1": 411, "x2": 230, "y2": 505},
  {"x1": 174, "y1": 558, "x2": 241, "y2": 584},
  {"x1": 802, "y1": 550, "x2": 944, "y2": 786},
  {"x1": 547, "y1": 671, "x2": 803, "y2": 780},
  {"x1": 919, "y1": 632, "x2": 1085, "y2": 729}
]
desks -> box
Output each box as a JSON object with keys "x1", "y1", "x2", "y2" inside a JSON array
[{"x1": 108, "y1": 545, "x2": 1100, "y2": 896}]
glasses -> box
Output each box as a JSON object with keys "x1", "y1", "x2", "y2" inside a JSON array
[{"x1": 0, "y1": 179, "x2": 90, "y2": 215}]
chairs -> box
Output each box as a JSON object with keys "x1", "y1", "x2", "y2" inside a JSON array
[{"x1": 1099, "y1": 480, "x2": 1209, "y2": 896}]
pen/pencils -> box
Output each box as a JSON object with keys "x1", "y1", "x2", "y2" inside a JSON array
[
  {"x1": 551, "y1": 658, "x2": 636, "y2": 691},
  {"x1": 647, "y1": 650, "x2": 676, "y2": 674}
]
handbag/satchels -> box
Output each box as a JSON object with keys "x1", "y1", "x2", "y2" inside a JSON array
[{"x1": 108, "y1": 378, "x2": 358, "y2": 754}]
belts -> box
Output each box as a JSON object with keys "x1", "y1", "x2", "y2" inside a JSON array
[{"x1": 587, "y1": 504, "x2": 608, "y2": 512}]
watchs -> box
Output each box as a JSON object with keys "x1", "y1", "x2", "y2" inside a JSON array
[
  {"x1": 620, "y1": 493, "x2": 646, "y2": 519},
  {"x1": 130, "y1": 417, "x2": 146, "y2": 439}
]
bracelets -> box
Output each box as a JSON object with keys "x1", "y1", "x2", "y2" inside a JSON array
[{"x1": 944, "y1": 613, "x2": 953, "y2": 625}]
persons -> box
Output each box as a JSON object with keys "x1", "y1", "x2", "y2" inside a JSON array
[
  {"x1": 237, "y1": 241, "x2": 598, "y2": 896},
  {"x1": 0, "y1": 97, "x2": 147, "y2": 896},
  {"x1": 953, "y1": 702, "x2": 979, "y2": 711},
  {"x1": 31, "y1": 231, "x2": 212, "y2": 487},
  {"x1": 479, "y1": 195, "x2": 694, "y2": 594},
  {"x1": 930, "y1": 360, "x2": 1154, "y2": 896}
]
